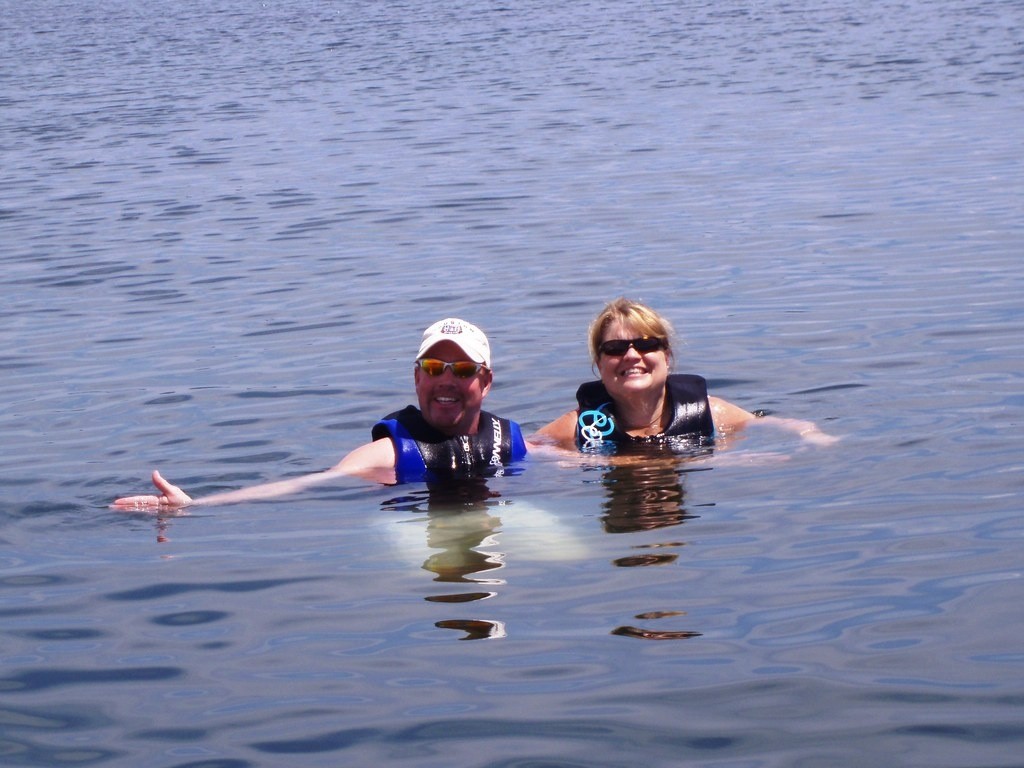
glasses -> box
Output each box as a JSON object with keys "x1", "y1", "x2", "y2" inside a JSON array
[
  {"x1": 596, "y1": 335, "x2": 669, "y2": 359},
  {"x1": 416, "y1": 356, "x2": 484, "y2": 378}
]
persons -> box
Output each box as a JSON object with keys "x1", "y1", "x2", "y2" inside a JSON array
[
  {"x1": 114, "y1": 317, "x2": 533, "y2": 508},
  {"x1": 537, "y1": 297, "x2": 758, "y2": 438}
]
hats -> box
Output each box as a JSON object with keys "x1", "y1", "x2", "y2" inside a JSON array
[{"x1": 413, "y1": 318, "x2": 491, "y2": 369}]
